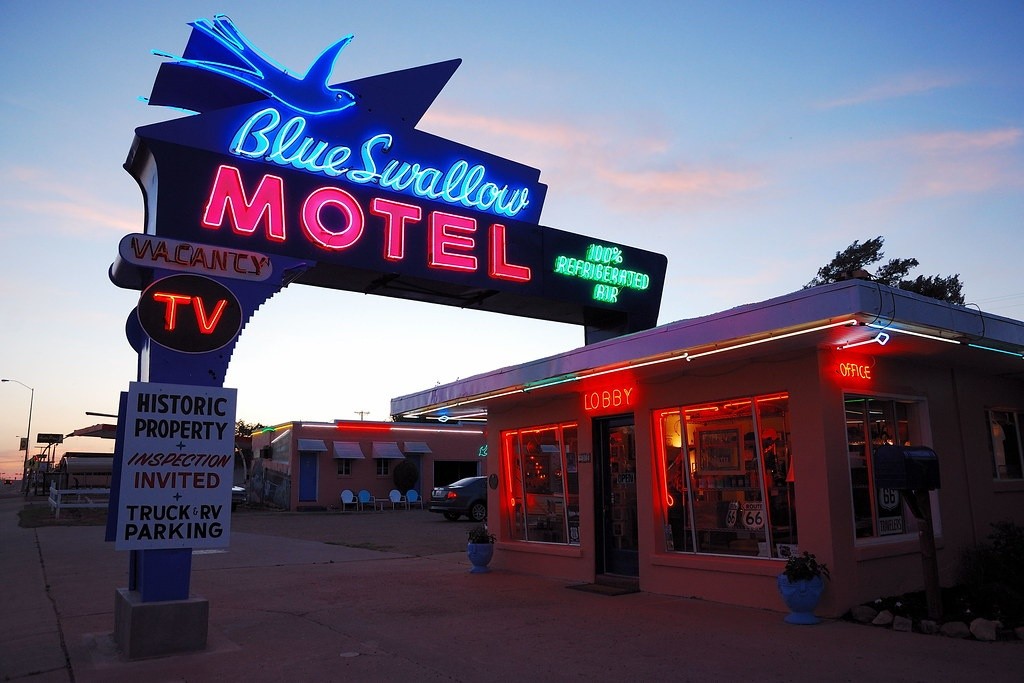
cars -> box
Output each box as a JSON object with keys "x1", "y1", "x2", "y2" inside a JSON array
[
  {"x1": 428, "y1": 476, "x2": 487, "y2": 522},
  {"x1": 232, "y1": 484, "x2": 248, "y2": 513}
]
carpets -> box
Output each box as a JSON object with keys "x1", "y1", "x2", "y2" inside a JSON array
[{"x1": 565, "y1": 583, "x2": 637, "y2": 597}]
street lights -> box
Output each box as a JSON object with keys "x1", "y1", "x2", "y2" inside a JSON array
[
  {"x1": 15, "y1": 435, "x2": 31, "y2": 487},
  {"x1": 0, "y1": 377, "x2": 34, "y2": 494}
]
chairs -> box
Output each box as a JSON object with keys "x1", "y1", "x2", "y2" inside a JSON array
[
  {"x1": 358, "y1": 490, "x2": 376, "y2": 511},
  {"x1": 340, "y1": 490, "x2": 358, "y2": 511},
  {"x1": 389, "y1": 490, "x2": 407, "y2": 511},
  {"x1": 406, "y1": 490, "x2": 423, "y2": 510}
]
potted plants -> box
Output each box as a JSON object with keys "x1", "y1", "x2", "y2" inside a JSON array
[
  {"x1": 777, "y1": 545, "x2": 831, "y2": 624},
  {"x1": 467, "y1": 527, "x2": 497, "y2": 573}
]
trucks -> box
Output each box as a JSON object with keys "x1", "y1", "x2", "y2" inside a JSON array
[{"x1": 516, "y1": 472, "x2": 625, "y2": 523}]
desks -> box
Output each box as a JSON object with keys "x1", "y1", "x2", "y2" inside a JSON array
[{"x1": 375, "y1": 499, "x2": 389, "y2": 513}]
